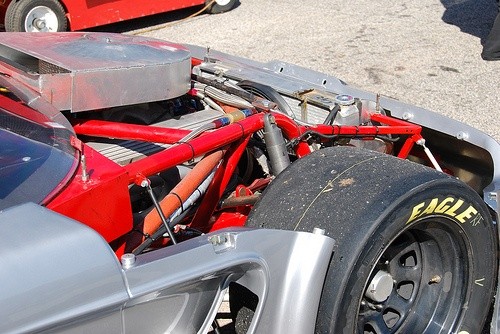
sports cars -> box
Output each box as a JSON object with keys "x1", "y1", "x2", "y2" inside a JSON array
[{"x1": 0, "y1": 27, "x2": 499, "y2": 333}]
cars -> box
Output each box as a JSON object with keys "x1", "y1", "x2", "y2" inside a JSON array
[{"x1": 0, "y1": 0, "x2": 242, "y2": 38}]
